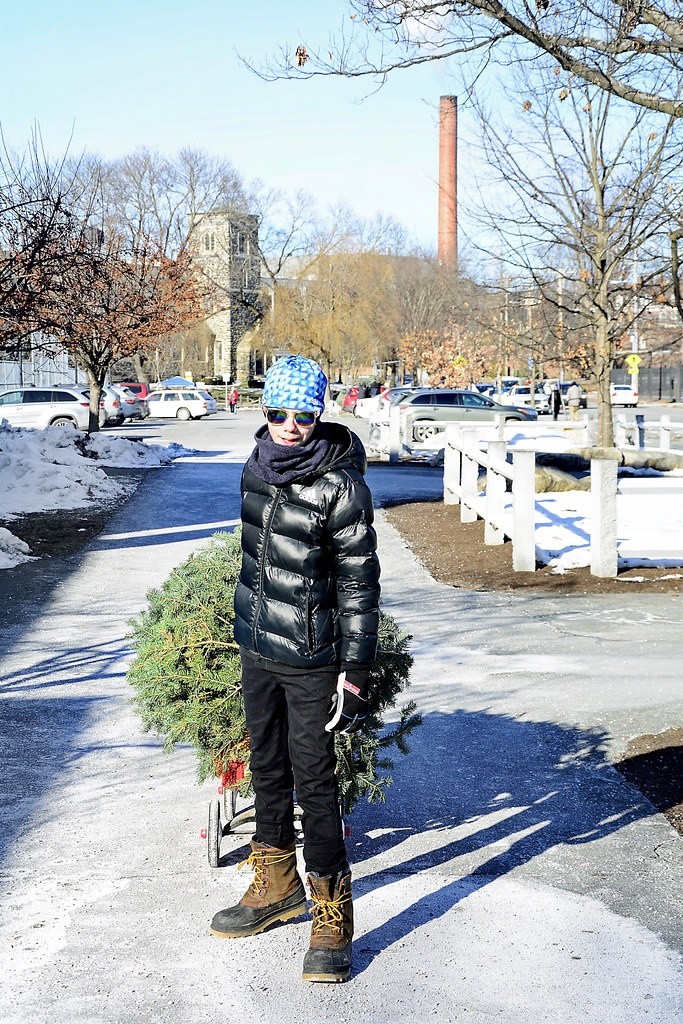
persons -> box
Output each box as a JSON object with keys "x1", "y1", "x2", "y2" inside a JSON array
[
  {"x1": 567, "y1": 381, "x2": 581, "y2": 421},
  {"x1": 365, "y1": 378, "x2": 384, "y2": 398},
  {"x1": 212, "y1": 354, "x2": 380, "y2": 982},
  {"x1": 548, "y1": 385, "x2": 561, "y2": 421},
  {"x1": 205, "y1": 389, "x2": 212, "y2": 397},
  {"x1": 228, "y1": 388, "x2": 239, "y2": 414}
]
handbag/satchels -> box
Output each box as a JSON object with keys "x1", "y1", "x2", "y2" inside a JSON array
[{"x1": 548, "y1": 405, "x2": 552, "y2": 413}]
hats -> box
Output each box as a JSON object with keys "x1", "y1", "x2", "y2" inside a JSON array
[{"x1": 261, "y1": 354, "x2": 328, "y2": 419}]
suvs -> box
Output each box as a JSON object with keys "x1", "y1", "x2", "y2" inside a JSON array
[
  {"x1": 109, "y1": 386, "x2": 141, "y2": 425},
  {"x1": 117, "y1": 382, "x2": 147, "y2": 398},
  {"x1": 390, "y1": 390, "x2": 538, "y2": 444},
  {"x1": 64, "y1": 386, "x2": 121, "y2": 424}
]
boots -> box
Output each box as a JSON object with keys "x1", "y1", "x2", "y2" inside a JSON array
[
  {"x1": 209, "y1": 833, "x2": 308, "y2": 939},
  {"x1": 302, "y1": 869, "x2": 354, "y2": 981}
]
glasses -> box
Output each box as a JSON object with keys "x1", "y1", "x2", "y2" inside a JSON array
[{"x1": 264, "y1": 408, "x2": 317, "y2": 428}]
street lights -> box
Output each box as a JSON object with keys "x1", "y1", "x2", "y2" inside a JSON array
[
  {"x1": 611, "y1": 280, "x2": 638, "y2": 394},
  {"x1": 509, "y1": 301, "x2": 536, "y2": 409}
]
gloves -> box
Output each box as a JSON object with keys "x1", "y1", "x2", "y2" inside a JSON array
[{"x1": 325, "y1": 670, "x2": 369, "y2": 736}]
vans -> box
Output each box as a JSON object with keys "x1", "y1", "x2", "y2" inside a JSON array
[{"x1": 493, "y1": 376, "x2": 521, "y2": 390}]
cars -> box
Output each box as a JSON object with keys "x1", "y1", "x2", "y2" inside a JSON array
[
  {"x1": 0, "y1": 388, "x2": 106, "y2": 430},
  {"x1": 464, "y1": 384, "x2": 497, "y2": 407},
  {"x1": 559, "y1": 382, "x2": 587, "y2": 408},
  {"x1": 123, "y1": 398, "x2": 149, "y2": 423},
  {"x1": 142, "y1": 390, "x2": 217, "y2": 421},
  {"x1": 610, "y1": 385, "x2": 639, "y2": 408},
  {"x1": 328, "y1": 384, "x2": 447, "y2": 428},
  {"x1": 495, "y1": 387, "x2": 512, "y2": 395}
]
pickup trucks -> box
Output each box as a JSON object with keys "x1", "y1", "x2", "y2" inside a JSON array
[{"x1": 502, "y1": 386, "x2": 550, "y2": 414}]
